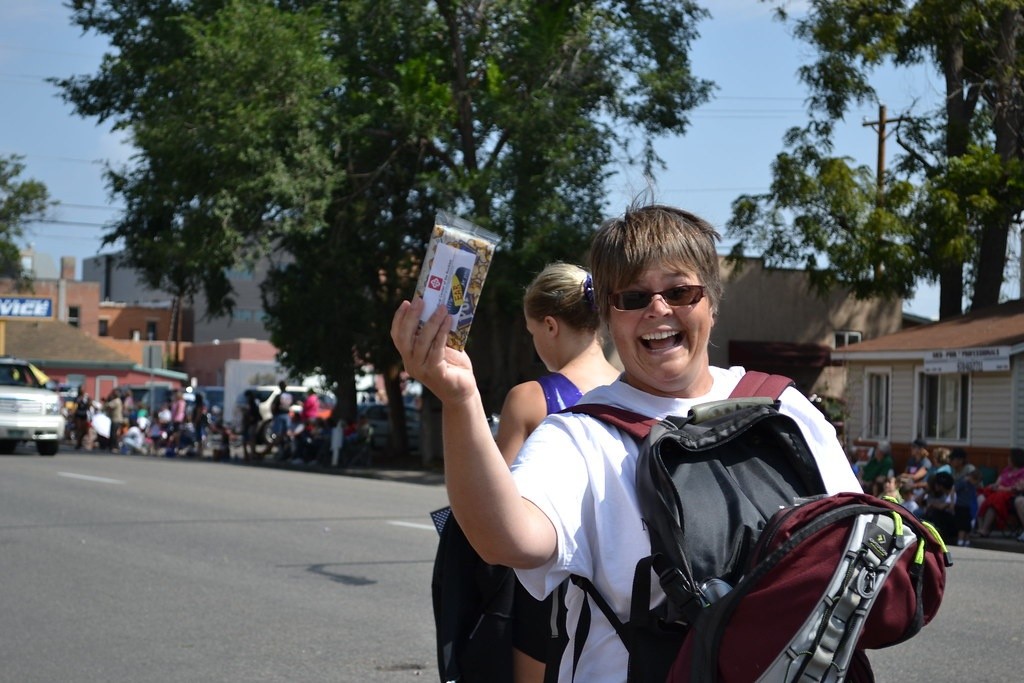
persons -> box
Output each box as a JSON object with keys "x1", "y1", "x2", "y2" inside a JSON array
[
  {"x1": 391, "y1": 176, "x2": 1024, "y2": 683},
  {"x1": 66, "y1": 380, "x2": 368, "y2": 468}
]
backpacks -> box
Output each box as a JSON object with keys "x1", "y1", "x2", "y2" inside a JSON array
[{"x1": 564, "y1": 371, "x2": 953, "y2": 683}]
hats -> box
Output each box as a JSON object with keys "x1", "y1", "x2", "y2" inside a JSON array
[{"x1": 914, "y1": 439, "x2": 928, "y2": 448}]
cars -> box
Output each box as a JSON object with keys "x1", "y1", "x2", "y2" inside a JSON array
[{"x1": 114, "y1": 379, "x2": 421, "y2": 472}]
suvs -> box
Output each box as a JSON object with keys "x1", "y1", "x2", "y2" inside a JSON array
[{"x1": 0, "y1": 353, "x2": 67, "y2": 457}]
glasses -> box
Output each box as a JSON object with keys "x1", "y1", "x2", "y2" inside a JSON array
[{"x1": 607, "y1": 285, "x2": 708, "y2": 311}]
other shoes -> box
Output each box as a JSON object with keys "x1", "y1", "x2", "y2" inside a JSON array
[
  {"x1": 1017, "y1": 532, "x2": 1024, "y2": 542},
  {"x1": 976, "y1": 529, "x2": 989, "y2": 537},
  {"x1": 957, "y1": 539, "x2": 964, "y2": 546},
  {"x1": 965, "y1": 540, "x2": 971, "y2": 546}
]
